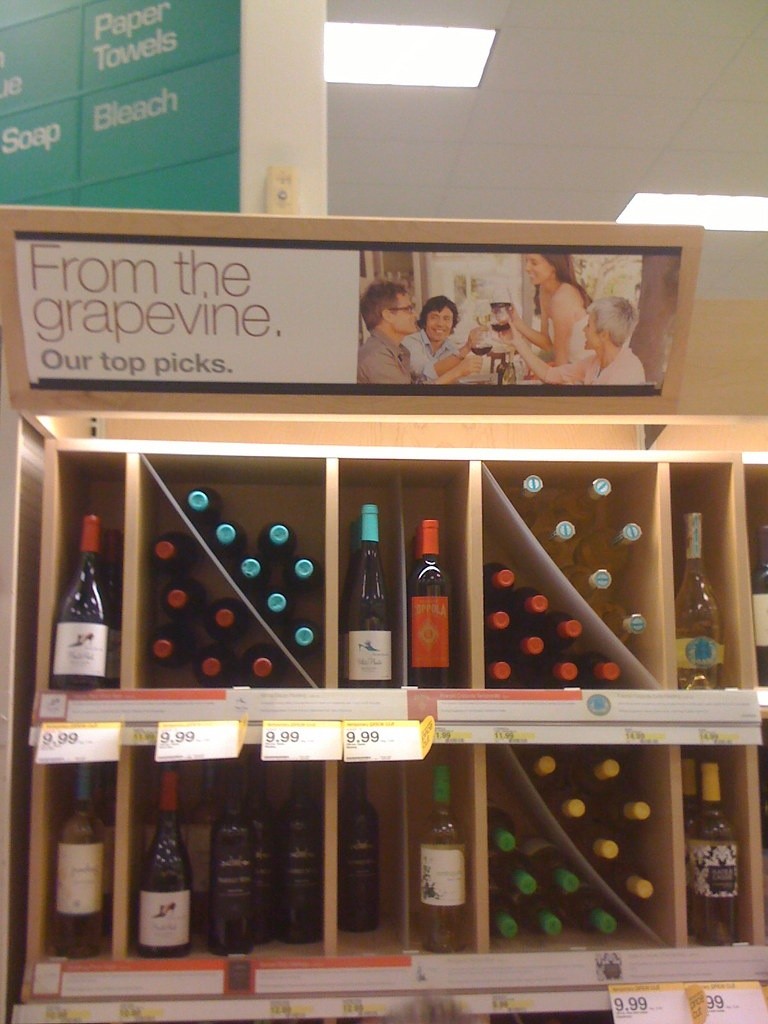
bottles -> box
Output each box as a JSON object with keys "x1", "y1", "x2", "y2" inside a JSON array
[
  {"x1": 208, "y1": 750, "x2": 322, "y2": 957},
  {"x1": 407, "y1": 520, "x2": 453, "y2": 689},
  {"x1": 339, "y1": 761, "x2": 381, "y2": 932},
  {"x1": 54, "y1": 763, "x2": 115, "y2": 959},
  {"x1": 488, "y1": 749, "x2": 654, "y2": 939},
  {"x1": 674, "y1": 513, "x2": 726, "y2": 690},
  {"x1": 419, "y1": 763, "x2": 469, "y2": 954},
  {"x1": 495, "y1": 352, "x2": 515, "y2": 385},
  {"x1": 682, "y1": 756, "x2": 738, "y2": 948},
  {"x1": 52, "y1": 514, "x2": 120, "y2": 689},
  {"x1": 484, "y1": 474, "x2": 645, "y2": 689},
  {"x1": 751, "y1": 524, "x2": 768, "y2": 685},
  {"x1": 136, "y1": 765, "x2": 195, "y2": 956},
  {"x1": 148, "y1": 485, "x2": 394, "y2": 687}
]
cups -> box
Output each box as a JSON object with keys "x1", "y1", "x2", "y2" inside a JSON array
[{"x1": 471, "y1": 284, "x2": 514, "y2": 355}]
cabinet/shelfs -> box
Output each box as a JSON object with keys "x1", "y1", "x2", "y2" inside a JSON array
[{"x1": 21, "y1": 436, "x2": 767, "y2": 998}]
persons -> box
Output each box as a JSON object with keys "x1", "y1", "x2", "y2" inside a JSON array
[
  {"x1": 400, "y1": 296, "x2": 490, "y2": 381},
  {"x1": 357, "y1": 280, "x2": 482, "y2": 385},
  {"x1": 496, "y1": 253, "x2": 596, "y2": 367},
  {"x1": 498, "y1": 297, "x2": 645, "y2": 385}
]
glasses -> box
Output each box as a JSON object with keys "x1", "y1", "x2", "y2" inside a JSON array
[{"x1": 380, "y1": 302, "x2": 417, "y2": 318}]
